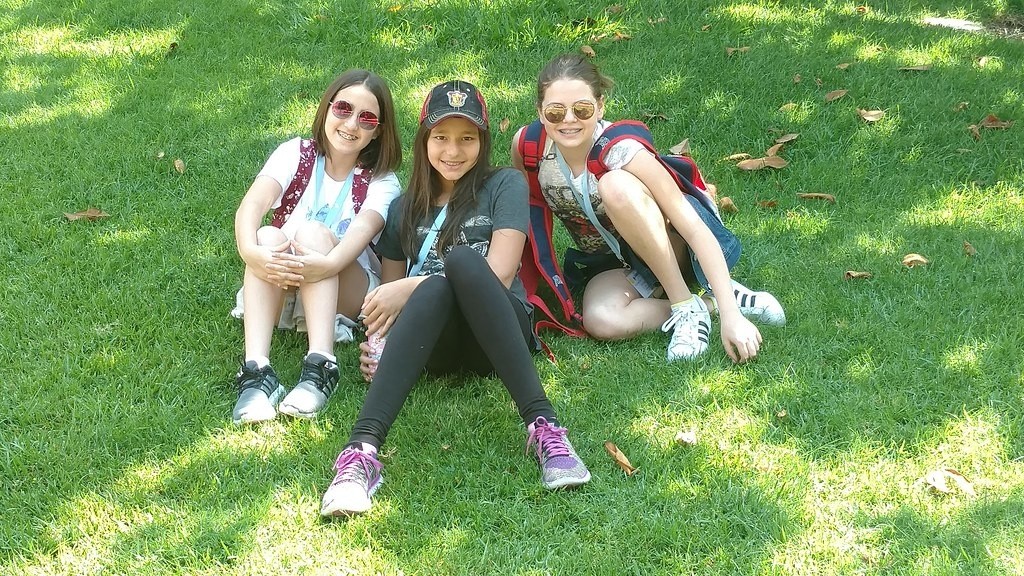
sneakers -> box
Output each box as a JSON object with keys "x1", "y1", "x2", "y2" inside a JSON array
[
  {"x1": 229, "y1": 355, "x2": 287, "y2": 425},
  {"x1": 706, "y1": 278, "x2": 787, "y2": 327},
  {"x1": 527, "y1": 415, "x2": 592, "y2": 491},
  {"x1": 321, "y1": 442, "x2": 386, "y2": 516},
  {"x1": 661, "y1": 294, "x2": 711, "y2": 359},
  {"x1": 279, "y1": 352, "x2": 342, "y2": 419}
]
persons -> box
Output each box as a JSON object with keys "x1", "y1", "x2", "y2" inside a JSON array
[
  {"x1": 512, "y1": 56, "x2": 787, "y2": 367},
  {"x1": 231, "y1": 71, "x2": 402, "y2": 426},
  {"x1": 320, "y1": 80, "x2": 592, "y2": 518}
]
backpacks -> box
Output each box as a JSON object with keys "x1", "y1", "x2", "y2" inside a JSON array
[{"x1": 517, "y1": 119, "x2": 719, "y2": 362}]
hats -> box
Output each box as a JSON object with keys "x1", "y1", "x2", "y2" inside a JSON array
[{"x1": 420, "y1": 80, "x2": 489, "y2": 132}]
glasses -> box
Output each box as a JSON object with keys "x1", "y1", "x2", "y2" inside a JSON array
[
  {"x1": 329, "y1": 100, "x2": 381, "y2": 130},
  {"x1": 541, "y1": 99, "x2": 597, "y2": 124}
]
